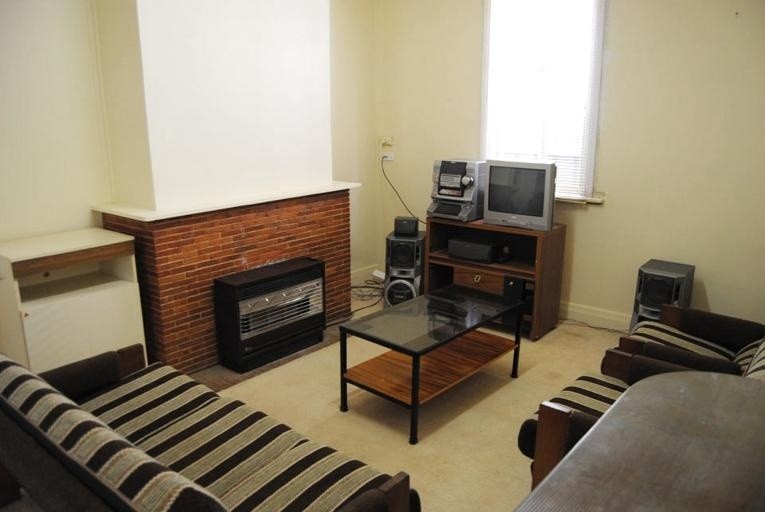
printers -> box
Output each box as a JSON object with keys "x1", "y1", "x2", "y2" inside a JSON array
[{"x1": 425, "y1": 158, "x2": 490, "y2": 233}]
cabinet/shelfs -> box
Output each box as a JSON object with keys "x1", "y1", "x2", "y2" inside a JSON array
[
  {"x1": 1, "y1": 227, "x2": 149, "y2": 374},
  {"x1": 423, "y1": 215, "x2": 568, "y2": 342}
]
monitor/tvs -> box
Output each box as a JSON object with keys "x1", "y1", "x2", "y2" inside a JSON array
[{"x1": 483, "y1": 159, "x2": 558, "y2": 231}]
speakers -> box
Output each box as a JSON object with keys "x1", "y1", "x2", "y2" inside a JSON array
[
  {"x1": 383, "y1": 231, "x2": 426, "y2": 308},
  {"x1": 628, "y1": 258, "x2": 696, "y2": 336},
  {"x1": 394, "y1": 216, "x2": 419, "y2": 236}
]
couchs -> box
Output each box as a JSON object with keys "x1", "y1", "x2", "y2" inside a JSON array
[{"x1": 0, "y1": 343, "x2": 422, "y2": 511}]
